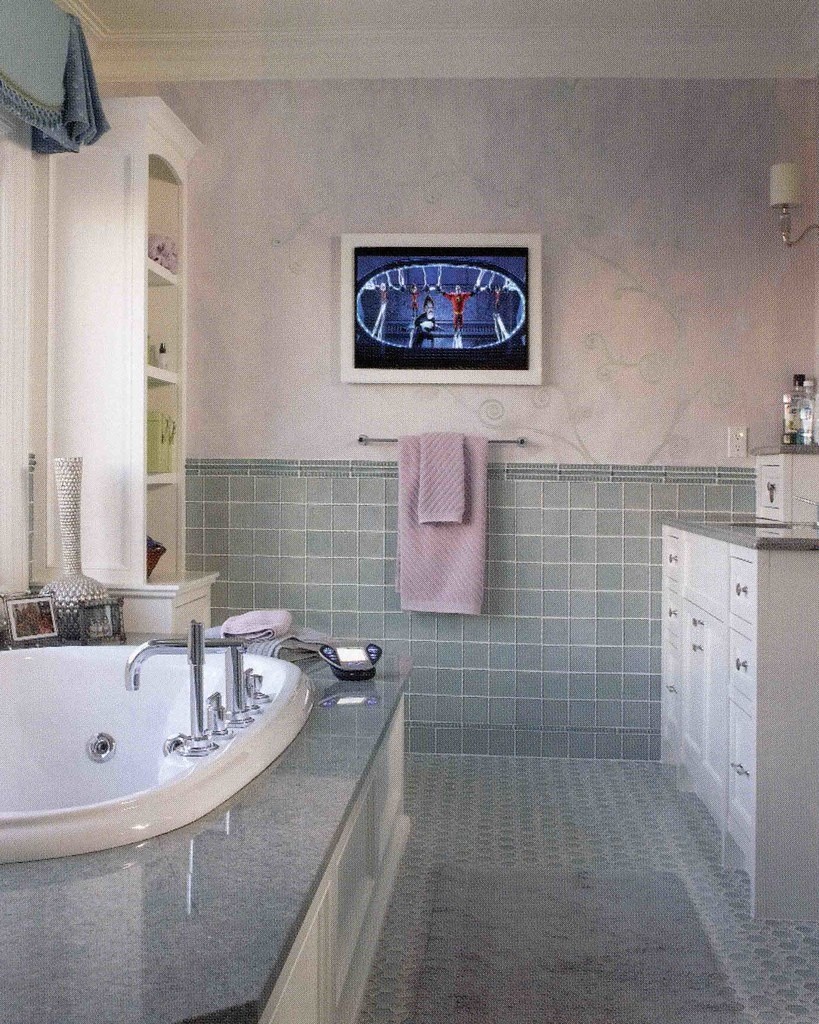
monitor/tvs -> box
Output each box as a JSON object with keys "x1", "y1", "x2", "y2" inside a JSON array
[{"x1": 341, "y1": 233, "x2": 544, "y2": 386}]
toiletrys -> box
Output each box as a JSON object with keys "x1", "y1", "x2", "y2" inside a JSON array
[{"x1": 780, "y1": 372, "x2": 819, "y2": 446}]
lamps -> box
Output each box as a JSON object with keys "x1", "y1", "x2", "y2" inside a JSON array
[{"x1": 768, "y1": 159, "x2": 819, "y2": 246}]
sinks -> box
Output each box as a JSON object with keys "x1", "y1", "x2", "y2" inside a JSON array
[{"x1": 0, "y1": 638, "x2": 316, "y2": 867}]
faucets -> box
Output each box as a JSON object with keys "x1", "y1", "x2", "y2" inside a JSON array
[{"x1": 124, "y1": 636, "x2": 254, "y2": 727}]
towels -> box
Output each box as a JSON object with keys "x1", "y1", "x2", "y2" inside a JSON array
[
  {"x1": 418, "y1": 429, "x2": 468, "y2": 526},
  {"x1": 393, "y1": 431, "x2": 492, "y2": 621},
  {"x1": 203, "y1": 623, "x2": 334, "y2": 662},
  {"x1": 219, "y1": 609, "x2": 293, "y2": 640}
]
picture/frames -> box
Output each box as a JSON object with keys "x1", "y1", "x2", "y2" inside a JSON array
[
  {"x1": 77, "y1": 597, "x2": 127, "y2": 645},
  {"x1": 4, "y1": 593, "x2": 59, "y2": 642}
]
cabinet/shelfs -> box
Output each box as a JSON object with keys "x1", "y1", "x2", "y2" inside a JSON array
[
  {"x1": 658, "y1": 519, "x2": 819, "y2": 926},
  {"x1": 266, "y1": 693, "x2": 412, "y2": 1024},
  {"x1": 44, "y1": 94, "x2": 221, "y2": 633}
]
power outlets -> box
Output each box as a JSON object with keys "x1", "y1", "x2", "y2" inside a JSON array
[{"x1": 729, "y1": 425, "x2": 748, "y2": 457}]
